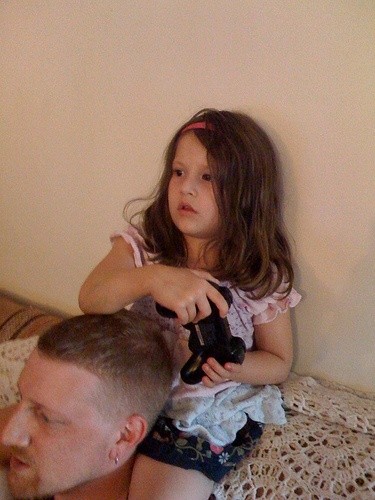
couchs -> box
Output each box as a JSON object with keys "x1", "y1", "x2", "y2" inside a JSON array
[{"x1": 0, "y1": 289, "x2": 374, "y2": 500}]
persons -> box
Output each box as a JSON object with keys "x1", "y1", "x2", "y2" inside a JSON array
[
  {"x1": 3, "y1": 314, "x2": 173, "y2": 500},
  {"x1": 0, "y1": 109, "x2": 302, "y2": 500}
]
